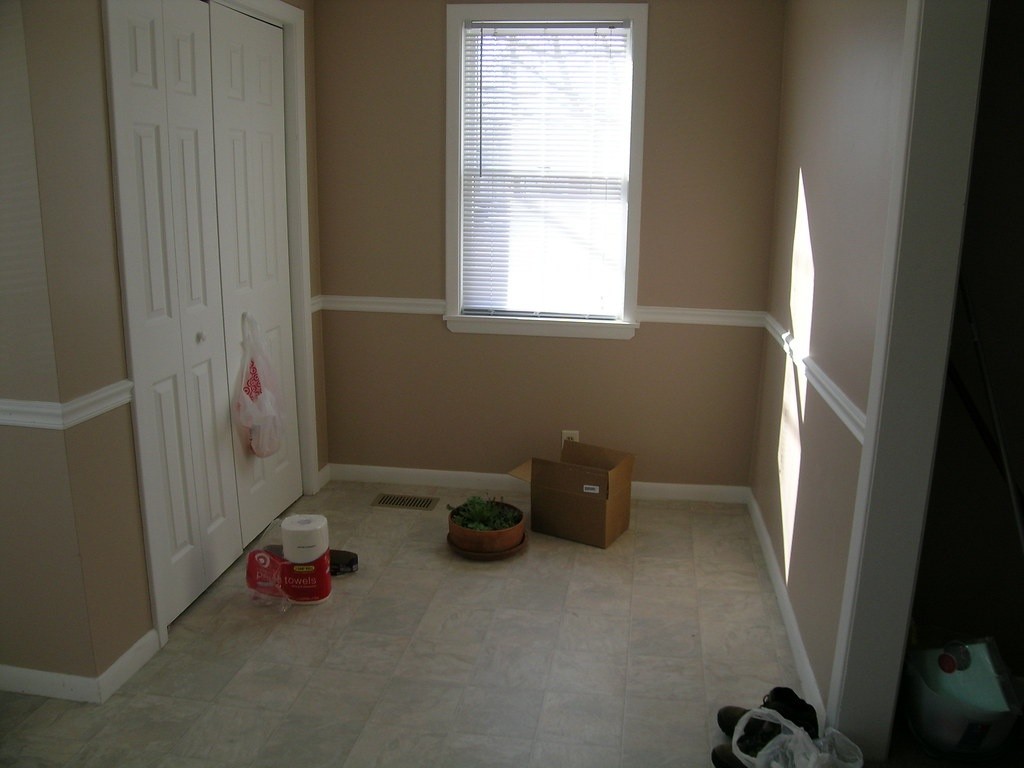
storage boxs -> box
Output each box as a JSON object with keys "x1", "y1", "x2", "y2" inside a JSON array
[{"x1": 507, "y1": 440, "x2": 639, "y2": 550}]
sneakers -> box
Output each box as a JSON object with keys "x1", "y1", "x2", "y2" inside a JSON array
[
  {"x1": 711, "y1": 703, "x2": 818, "y2": 768},
  {"x1": 717, "y1": 687, "x2": 800, "y2": 740}
]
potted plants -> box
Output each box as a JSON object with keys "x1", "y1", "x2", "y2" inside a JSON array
[{"x1": 446, "y1": 496, "x2": 525, "y2": 553}]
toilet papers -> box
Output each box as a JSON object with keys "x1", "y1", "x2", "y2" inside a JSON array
[{"x1": 280, "y1": 514, "x2": 333, "y2": 605}]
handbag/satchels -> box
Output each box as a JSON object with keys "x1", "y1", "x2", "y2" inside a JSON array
[
  {"x1": 233, "y1": 311, "x2": 284, "y2": 457},
  {"x1": 732, "y1": 707, "x2": 864, "y2": 768}
]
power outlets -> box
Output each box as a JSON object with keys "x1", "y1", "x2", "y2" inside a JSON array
[{"x1": 562, "y1": 431, "x2": 580, "y2": 449}]
